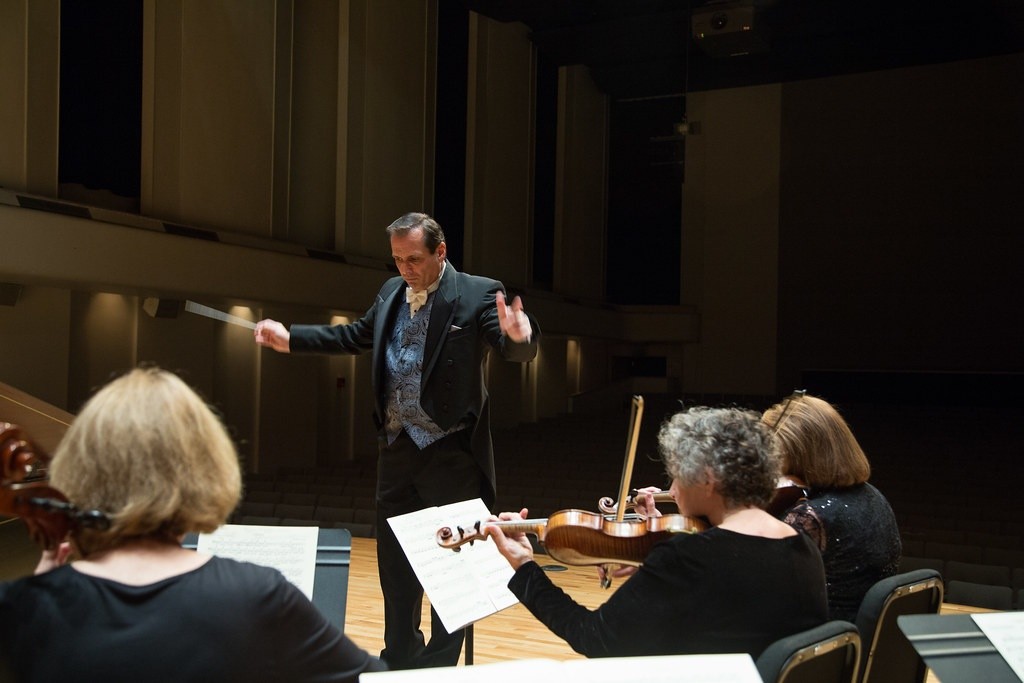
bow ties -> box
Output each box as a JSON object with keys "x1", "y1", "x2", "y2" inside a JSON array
[{"x1": 405, "y1": 287, "x2": 428, "y2": 306}]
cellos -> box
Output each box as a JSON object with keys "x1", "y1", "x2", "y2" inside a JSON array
[{"x1": 0, "y1": 420, "x2": 109, "y2": 550}]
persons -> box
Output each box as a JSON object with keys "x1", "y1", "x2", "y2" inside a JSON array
[
  {"x1": 486, "y1": 404, "x2": 833, "y2": 663},
  {"x1": 0, "y1": 366, "x2": 386, "y2": 683},
  {"x1": 632, "y1": 393, "x2": 902, "y2": 625},
  {"x1": 252, "y1": 212, "x2": 541, "y2": 669}
]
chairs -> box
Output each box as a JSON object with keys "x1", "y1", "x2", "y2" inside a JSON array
[{"x1": 756, "y1": 570, "x2": 944, "y2": 683}]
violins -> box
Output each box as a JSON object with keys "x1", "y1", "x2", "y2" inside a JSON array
[
  {"x1": 598, "y1": 477, "x2": 807, "y2": 514},
  {"x1": 436, "y1": 510, "x2": 709, "y2": 567}
]
